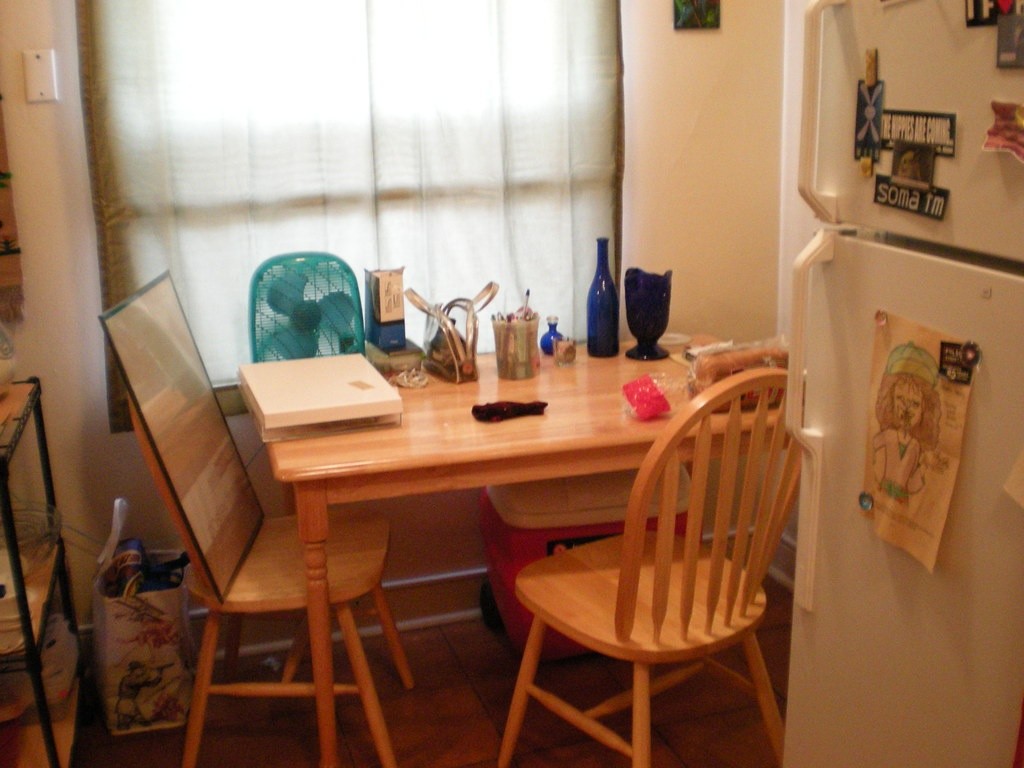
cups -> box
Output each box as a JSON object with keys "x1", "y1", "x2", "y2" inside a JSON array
[
  {"x1": 491, "y1": 317, "x2": 541, "y2": 380},
  {"x1": 624, "y1": 267, "x2": 674, "y2": 360},
  {"x1": 551, "y1": 336, "x2": 577, "y2": 367}
]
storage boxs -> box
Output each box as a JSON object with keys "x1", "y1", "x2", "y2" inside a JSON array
[{"x1": 480, "y1": 462, "x2": 702, "y2": 662}]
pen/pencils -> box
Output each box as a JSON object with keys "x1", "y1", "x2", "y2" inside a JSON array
[
  {"x1": 490, "y1": 312, "x2": 541, "y2": 379},
  {"x1": 523, "y1": 289, "x2": 530, "y2": 318}
]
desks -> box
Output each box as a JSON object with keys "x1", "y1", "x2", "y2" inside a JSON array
[{"x1": 268, "y1": 335, "x2": 792, "y2": 768}]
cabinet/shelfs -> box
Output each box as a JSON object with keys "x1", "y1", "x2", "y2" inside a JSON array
[{"x1": 0, "y1": 376, "x2": 90, "y2": 768}]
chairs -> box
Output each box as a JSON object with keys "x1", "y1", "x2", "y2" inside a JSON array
[
  {"x1": 494, "y1": 365, "x2": 802, "y2": 768},
  {"x1": 127, "y1": 378, "x2": 415, "y2": 767}
]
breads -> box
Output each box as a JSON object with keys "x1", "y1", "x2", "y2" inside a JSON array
[{"x1": 689, "y1": 347, "x2": 789, "y2": 413}]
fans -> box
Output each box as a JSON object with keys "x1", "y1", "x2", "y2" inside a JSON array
[{"x1": 248, "y1": 251, "x2": 367, "y2": 364}]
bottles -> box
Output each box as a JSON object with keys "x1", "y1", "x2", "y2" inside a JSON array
[
  {"x1": 585, "y1": 237, "x2": 620, "y2": 358},
  {"x1": 540, "y1": 316, "x2": 563, "y2": 355}
]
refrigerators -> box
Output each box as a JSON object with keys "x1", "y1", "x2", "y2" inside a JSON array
[{"x1": 784, "y1": 0, "x2": 1024, "y2": 768}]
